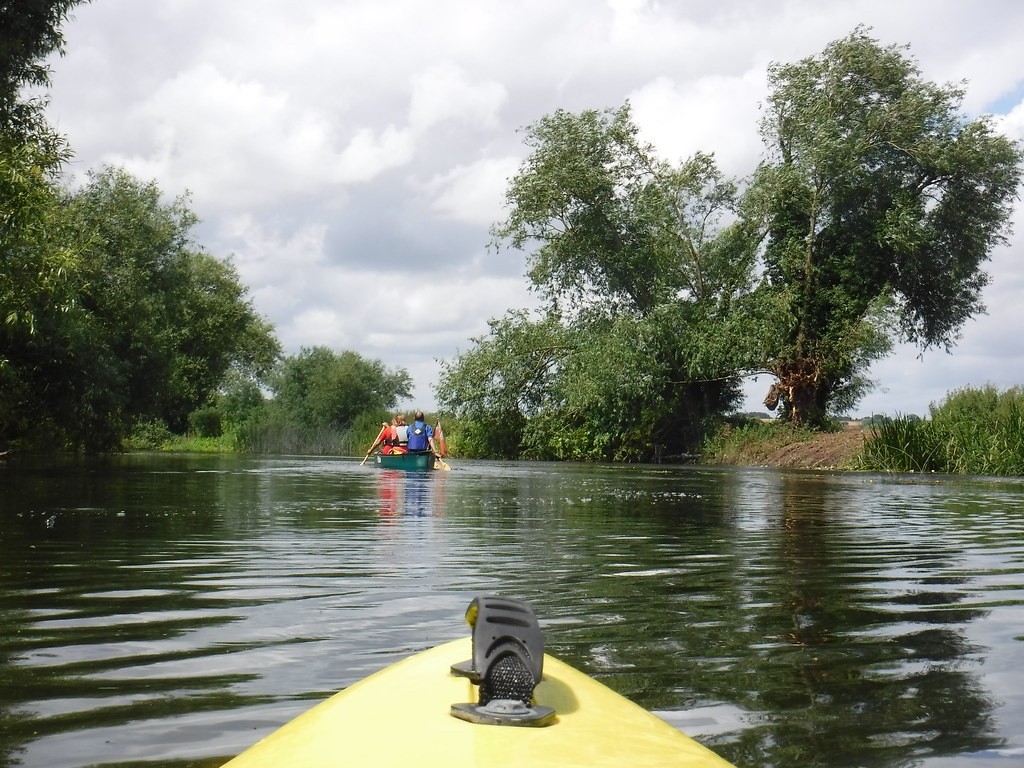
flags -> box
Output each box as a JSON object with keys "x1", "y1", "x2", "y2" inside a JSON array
[{"x1": 433, "y1": 422, "x2": 446, "y2": 455}]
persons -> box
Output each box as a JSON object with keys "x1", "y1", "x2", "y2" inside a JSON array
[{"x1": 366, "y1": 411, "x2": 442, "y2": 461}]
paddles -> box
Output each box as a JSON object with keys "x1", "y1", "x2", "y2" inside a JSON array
[
  {"x1": 360, "y1": 426, "x2": 386, "y2": 465},
  {"x1": 438, "y1": 458, "x2": 451, "y2": 472}
]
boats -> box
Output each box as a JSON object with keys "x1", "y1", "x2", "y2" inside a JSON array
[{"x1": 374, "y1": 450, "x2": 437, "y2": 471}]
